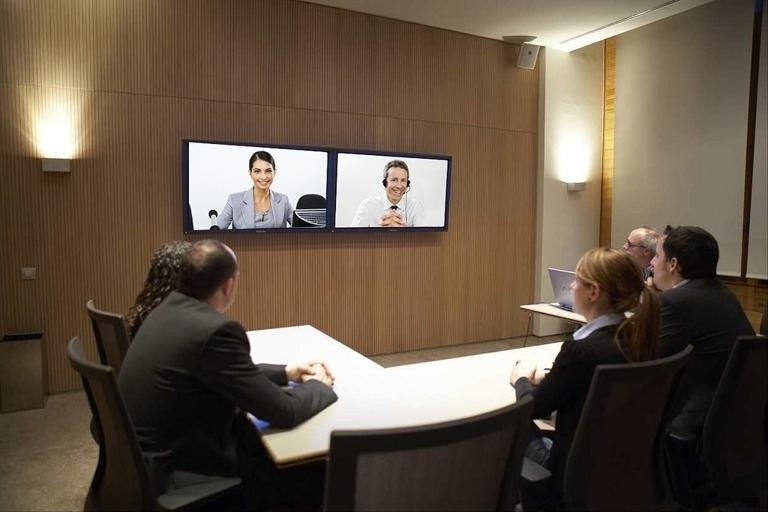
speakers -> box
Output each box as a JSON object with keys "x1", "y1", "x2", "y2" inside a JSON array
[{"x1": 515, "y1": 44, "x2": 540, "y2": 70}]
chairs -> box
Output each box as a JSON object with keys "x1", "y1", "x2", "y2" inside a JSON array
[
  {"x1": 696, "y1": 331, "x2": 767, "y2": 512},
  {"x1": 66, "y1": 334, "x2": 240, "y2": 509},
  {"x1": 557, "y1": 340, "x2": 703, "y2": 504},
  {"x1": 325, "y1": 392, "x2": 540, "y2": 511},
  {"x1": 85, "y1": 297, "x2": 128, "y2": 372}
]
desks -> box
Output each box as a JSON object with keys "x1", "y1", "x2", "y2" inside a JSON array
[
  {"x1": 230, "y1": 318, "x2": 574, "y2": 504},
  {"x1": 518, "y1": 300, "x2": 637, "y2": 341}
]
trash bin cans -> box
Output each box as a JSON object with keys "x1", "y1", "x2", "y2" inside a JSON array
[{"x1": 0, "y1": 332, "x2": 46, "y2": 413}]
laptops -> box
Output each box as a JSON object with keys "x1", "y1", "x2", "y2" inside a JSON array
[{"x1": 548, "y1": 268, "x2": 576, "y2": 311}]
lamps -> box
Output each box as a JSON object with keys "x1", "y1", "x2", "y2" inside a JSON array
[
  {"x1": 554, "y1": 130, "x2": 598, "y2": 194},
  {"x1": 41, "y1": 123, "x2": 73, "y2": 177}
]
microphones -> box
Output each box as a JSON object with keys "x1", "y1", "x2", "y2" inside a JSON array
[
  {"x1": 403, "y1": 186, "x2": 411, "y2": 194},
  {"x1": 209, "y1": 210, "x2": 221, "y2": 230}
]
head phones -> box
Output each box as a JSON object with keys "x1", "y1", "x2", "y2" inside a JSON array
[{"x1": 382, "y1": 165, "x2": 410, "y2": 187}]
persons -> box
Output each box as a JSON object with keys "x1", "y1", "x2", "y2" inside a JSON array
[
  {"x1": 507, "y1": 248, "x2": 660, "y2": 512},
  {"x1": 121, "y1": 242, "x2": 193, "y2": 341},
  {"x1": 351, "y1": 160, "x2": 426, "y2": 227},
  {"x1": 649, "y1": 226, "x2": 753, "y2": 502},
  {"x1": 619, "y1": 228, "x2": 654, "y2": 281},
  {"x1": 89, "y1": 240, "x2": 337, "y2": 485},
  {"x1": 217, "y1": 150, "x2": 294, "y2": 228}
]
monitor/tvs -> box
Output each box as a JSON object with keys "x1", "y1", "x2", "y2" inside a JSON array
[
  {"x1": 292, "y1": 208, "x2": 327, "y2": 227},
  {"x1": 181, "y1": 139, "x2": 333, "y2": 235},
  {"x1": 332, "y1": 149, "x2": 453, "y2": 233}
]
